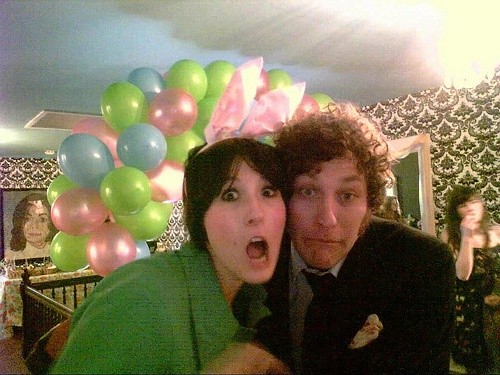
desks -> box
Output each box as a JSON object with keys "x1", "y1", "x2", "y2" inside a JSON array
[{"x1": 1, "y1": 270, "x2": 98, "y2": 341}]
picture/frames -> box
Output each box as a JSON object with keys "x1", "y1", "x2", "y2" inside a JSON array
[
  {"x1": 388, "y1": 134, "x2": 436, "y2": 237},
  {"x1": 0, "y1": 187, "x2": 58, "y2": 268}
]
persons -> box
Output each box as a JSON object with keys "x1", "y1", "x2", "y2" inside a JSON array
[
  {"x1": 7, "y1": 193, "x2": 59, "y2": 260},
  {"x1": 448, "y1": 186, "x2": 500, "y2": 375},
  {"x1": 47, "y1": 137, "x2": 293, "y2": 375},
  {"x1": 254, "y1": 116, "x2": 455, "y2": 375}
]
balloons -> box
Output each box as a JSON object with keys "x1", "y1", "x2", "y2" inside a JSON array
[{"x1": 47, "y1": 59, "x2": 339, "y2": 278}]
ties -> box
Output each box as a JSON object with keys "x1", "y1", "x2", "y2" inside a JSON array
[{"x1": 300, "y1": 268, "x2": 336, "y2": 375}]
